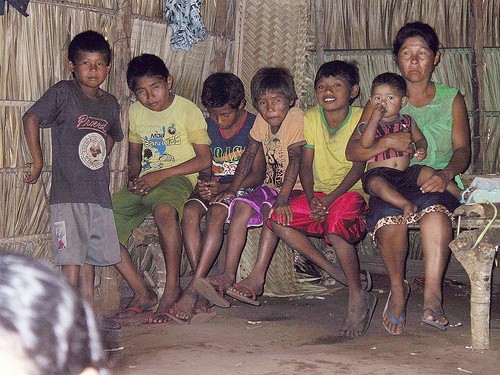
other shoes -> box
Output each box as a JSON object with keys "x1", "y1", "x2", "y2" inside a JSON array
[{"x1": 97, "y1": 319, "x2": 125, "y2": 332}]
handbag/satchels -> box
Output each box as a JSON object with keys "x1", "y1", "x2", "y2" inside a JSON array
[{"x1": 457, "y1": 177, "x2": 500, "y2": 249}]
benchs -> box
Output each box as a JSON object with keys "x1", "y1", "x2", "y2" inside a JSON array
[{"x1": 102, "y1": 174, "x2": 500, "y2": 351}]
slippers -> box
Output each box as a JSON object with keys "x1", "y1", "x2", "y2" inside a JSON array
[
  {"x1": 226, "y1": 283, "x2": 261, "y2": 307},
  {"x1": 167, "y1": 303, "x2": 191, "y2": 323},
  {"x1": 193, "y1": 277, "x2": 230, "y2": 307},
  {"x1": 111, "y1": 307, "x2": 144, "y2": 323},
  {"x1": 141, "y1": 312, "x2": 174, "y2": 326},
  {"x1": 382, "y1": 279, "x2": 411, "y2": 335},
  {"x1": 421, "y1": 307, "x2": 447, "y2": 331},
  {"x1": 344, "y1": 293, "x2": 377, "y2": 337},
  {"x1": 360, "y1": 269, "x2": 372, "y2": 292}
]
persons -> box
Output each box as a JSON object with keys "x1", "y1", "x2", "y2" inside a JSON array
[
  {"x1": 24, "y1": 30, "x2": 122, "y2": 334},
  {"x1": 209, "y1": 66, "x2": 304, "y2": 307},
  {"x1": 166, "y1": 73, "x2": 264, "y2": 325},
  {"x1": 344, "y1": 22, "x2": 473, "y2": 335},
  {"x1": 111, "y1": 52, "x2": 211, "y2": 326},
  {"x1": 361, "y1": 72, "x2": 477, "y2": 220},
  {"x1": 0, "y1": 252, "x2": 108, "y2": 375},
  {"x1": 266, "y1": 60, "x2": 377, "y2": 337}
]
